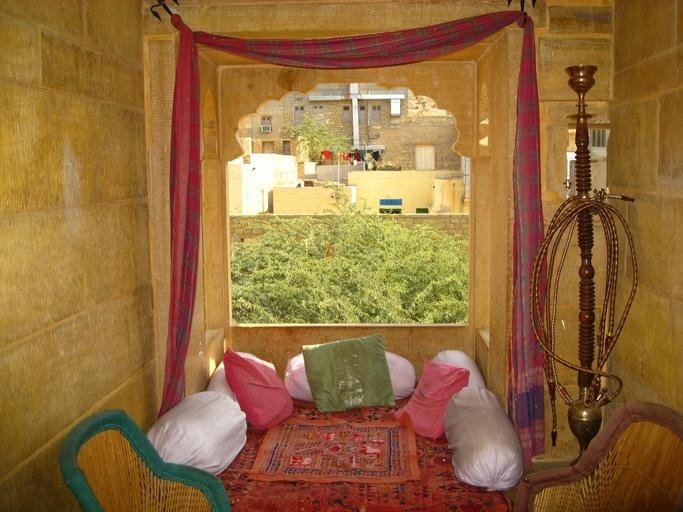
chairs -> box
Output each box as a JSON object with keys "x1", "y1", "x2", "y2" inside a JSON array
[
  {"x1": 59, "y1": 408, "x2": 233, "y2": 512},
  {"x1": 513, "y1": 402, "x2": 683, "y2": 512}
]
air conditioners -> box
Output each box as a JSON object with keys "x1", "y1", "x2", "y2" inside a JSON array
[{"x1": 261, "y1": 125, "x2": 271, "y2": 132}]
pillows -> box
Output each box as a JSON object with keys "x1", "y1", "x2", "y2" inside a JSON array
[
  {"x1": 432, "y1": 350, "x2": 486, "y2": 388},
  {"x1": 284, "y1": 351, "x2": 416, "y2": 401},
  {"x1": 206, "y1": 352, "x2": 275, "y2": 401},
  {"x1": 147, "y1": 392, "x2": 248, "y2": 480},
  {"x1": 223, "y1": 347, "x2": 294, "y2": 431},
  {"x1": 393, "y1": 358, "x2": 470, "y2": 441},
  {"x1": 302, "y1": 332, "x2": 396, "y2": 412},
  {"x1": 443, "y1": 387, "x2": 524, "y2": 492}
]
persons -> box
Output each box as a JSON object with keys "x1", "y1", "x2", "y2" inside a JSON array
[
  {"x1": 353, "y1": 148, "x2": 361, "y2": 161},
  {"x1": 364, "y1": 151, "x2": 372, "y2": 169},
  {"x1": 342, "y1": 150, "x2": 349, "y2": 160},
  {"x1": 320, "y1": 151, "x2": 326, "y2": 160},
  {"x1": 323, "y1": 149, "x2": 330, "y2": 160}
]
